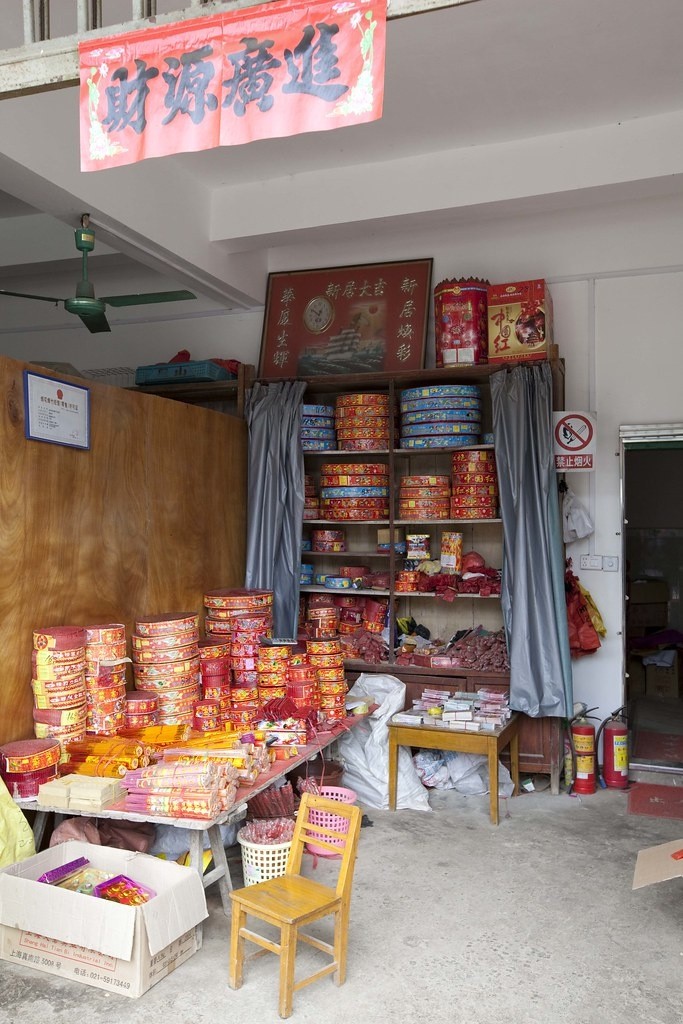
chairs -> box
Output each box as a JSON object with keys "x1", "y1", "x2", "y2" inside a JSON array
[{"x1": 229, "y1": 792, "x2": 362, "y2": 1016}]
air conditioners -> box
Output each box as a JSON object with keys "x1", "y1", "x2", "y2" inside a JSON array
[{"x1": 1, "y1": 839, "x2": 209, "y2": 1000}]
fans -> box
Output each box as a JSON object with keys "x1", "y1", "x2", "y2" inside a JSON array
[{"x1": 0, "y1": 212, "x2": 198, "y2": 334}]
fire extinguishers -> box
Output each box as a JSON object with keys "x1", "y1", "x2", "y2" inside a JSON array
[
  {"x1": 566, "y1": 705, "x2": 601, "y2": 797},
  {"x1": 595, "y1": 705, "x2": 632, "y2": 791}
]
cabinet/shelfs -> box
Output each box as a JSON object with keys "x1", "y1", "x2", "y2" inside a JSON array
[{"x1": 243, "y1": 339, "x2": 565, "y2": 797}]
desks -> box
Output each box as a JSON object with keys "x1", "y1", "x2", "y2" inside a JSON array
[
  {"x1": 16, "y1": 702, "x2": 380, "y2": 916},
  {"x1": 386, "y1": 708, "x2": 518, "y2": 826}
]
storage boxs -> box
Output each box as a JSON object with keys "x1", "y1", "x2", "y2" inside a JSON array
[
  {"x1": 628, "y1": 579, "x2": 670, "y2": 627},
  {"x1": 645, "y1": 650, "x2": 683, "y2": 698}
]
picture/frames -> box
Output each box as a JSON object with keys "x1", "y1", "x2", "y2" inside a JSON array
[{"x1": 257, "y1": 258, "x2": 433, "y2": 381}]
